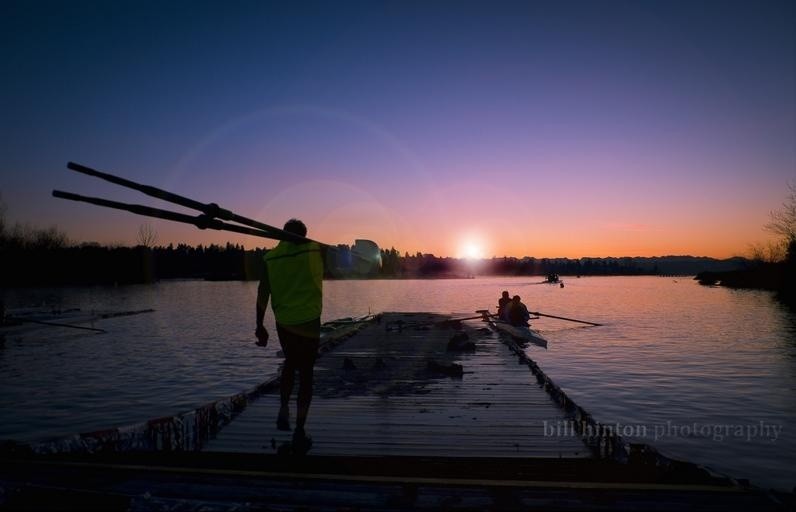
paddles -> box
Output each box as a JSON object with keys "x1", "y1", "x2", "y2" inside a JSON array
[
  {"x1": 529, "y1": 311, "x2": 599, "y2": 328},
  {"x1": 67, "y1": 163, "x2": 383, "y2": 269},
  {"x1": 51, "y1": 189, "x2": 382, "y2": 270},
  {"x1": 447, "y1": 313, "x2": 496, "y2": 323}
]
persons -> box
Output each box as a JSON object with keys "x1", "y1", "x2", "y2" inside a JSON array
[
  {"x1": 255, "y1": 217, "x2": 351, "y2": 450},
  {"x1": 498, "y1": 291, "x2": 530, "y2": 327}
]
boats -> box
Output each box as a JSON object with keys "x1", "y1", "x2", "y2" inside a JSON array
[
  {"x1": 0, "y1": 304, "x2": 155, "y2": 338},
  {"x1": 541, "y1": 280, "x2": 564, "y2": 284},
  {"x1": 486, "y1": 308, "x2": 548, "y2": 347},
  {"x1": 276, "y1": 312, "x2": 376, "y2": 358}
]
